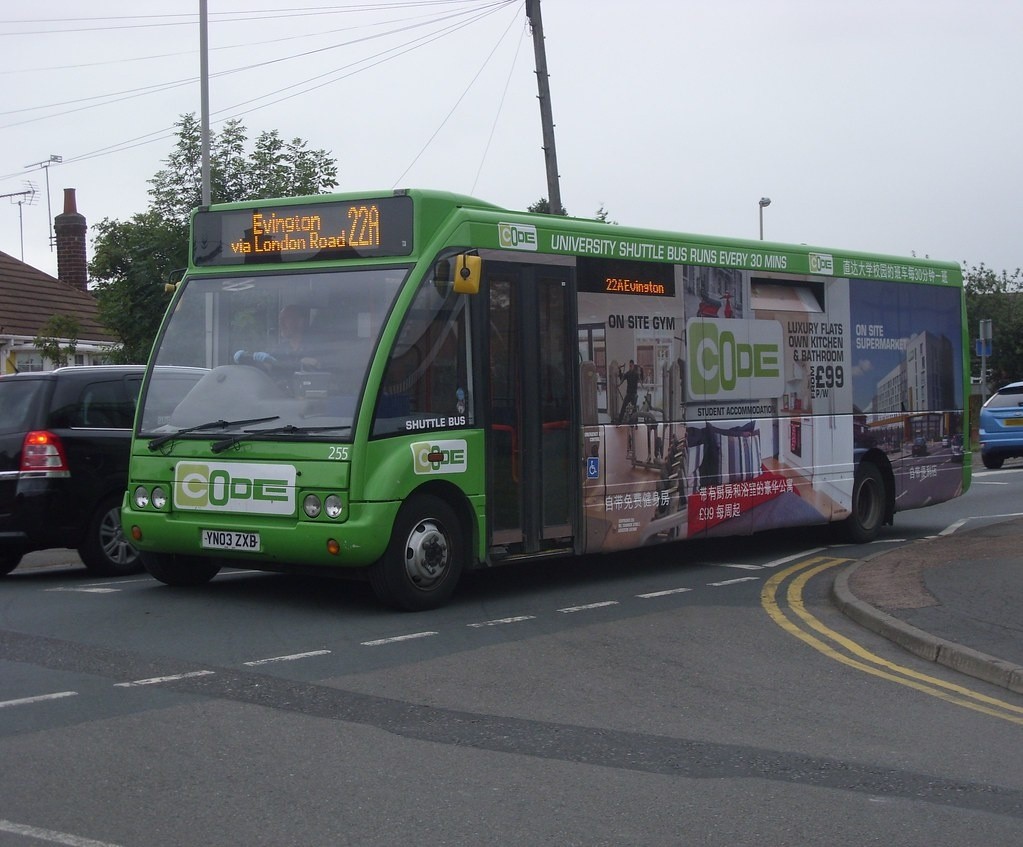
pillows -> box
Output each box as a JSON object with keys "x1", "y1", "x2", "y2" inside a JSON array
[{"x1": 685, "y1": 423, "x2": 764, "y2": 496}]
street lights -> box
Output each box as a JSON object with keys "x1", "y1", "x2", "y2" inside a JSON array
[{"x1": 757, "y1": 198, "x2": 771, "y2": 239}]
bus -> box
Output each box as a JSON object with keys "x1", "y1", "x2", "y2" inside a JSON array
[{"x1": 119, "y1": 188, "x2": 975, "y2": 610}]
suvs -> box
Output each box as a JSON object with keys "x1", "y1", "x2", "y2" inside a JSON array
[
  {"x1": 978, "y1": 380, "x2": 1023, "y2": 469},
  {"x1": 0, "y1": 364, "x2": 213, "y2": 576}
]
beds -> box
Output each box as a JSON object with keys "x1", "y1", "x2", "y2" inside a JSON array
[{"x1": 689, "y1": 463, "x2": 827, "y2": 540}]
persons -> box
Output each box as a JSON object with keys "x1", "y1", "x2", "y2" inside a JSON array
[
  {"x1": 613, "y1": 359, "x2": 643, "y2": 425},
  {"x1": 233, "y1": 303, "x2": 308, "y2": 385}
]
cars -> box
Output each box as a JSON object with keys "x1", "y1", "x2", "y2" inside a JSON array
[
  {"x1": 890, "y1": 441, "x2": 899, "y2": 452},
  {"x1": 910, "y1": 438, "x2": 929, "y2": 456},
  {"x1": 941, "y1": 435, "x2": 965, "y2": 461}
]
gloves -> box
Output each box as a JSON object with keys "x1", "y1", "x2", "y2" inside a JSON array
[
  {"x1": 253, "y1": 352, "x2": 271, "y2": 362},
  {"x1": 233, "y1": 349, "x2": 250, "y2": 364}
]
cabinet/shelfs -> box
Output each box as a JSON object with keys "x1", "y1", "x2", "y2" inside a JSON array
[{"x1": 775, "y1": 277, "x2": 853, "y2": 513}]
